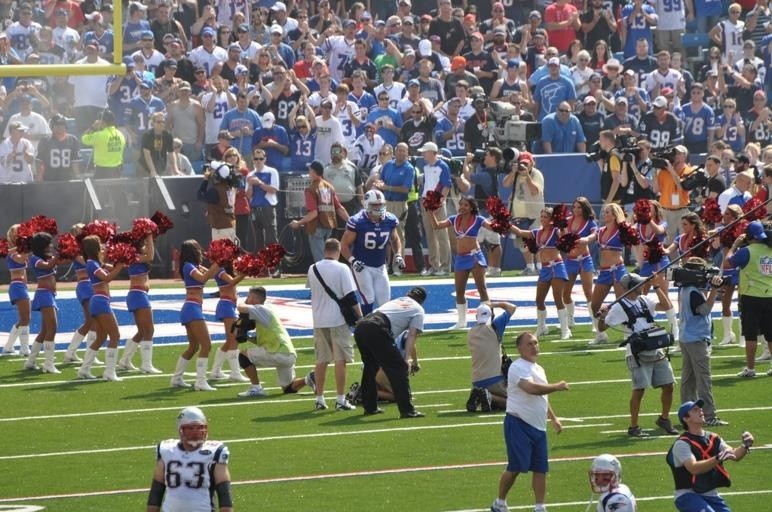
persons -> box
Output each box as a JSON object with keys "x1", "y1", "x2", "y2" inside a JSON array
[
  {"x1": 589, "y1": 453, "x2": 638, "y2": 512},
  {"x1": 491, "y1": 332, "x2": 569, "y2": 512},
  {"x1": 147, "y1": 408, "x2": 235, "y2": 512},
  {"x1": 1, "y1": 0, "x2": 771, "y2": 441},
  {"x1": 665, "y1": 401, "x2": 755, "y2": 512}
]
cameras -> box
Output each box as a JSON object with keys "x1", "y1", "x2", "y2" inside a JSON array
[
  {"x1": 723, "y1": 67, "x2": 727, "y2": 71},
  {"x1": 21, "y1": 88, "x2": 28, "y2": 92},
  {"x1": 514, "y1": 162, "x2": 528, "y2": 171},
  {"x1": 489, "y1": 306, "x2": 495, "y2": 320}
]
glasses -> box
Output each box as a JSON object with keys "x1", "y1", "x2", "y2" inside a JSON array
[{"x1": 254, "y1": 157, "x2": 265, "y2": 160}]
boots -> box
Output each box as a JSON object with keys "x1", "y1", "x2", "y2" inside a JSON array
[
  {"x1": 665, "y1": 308, "x2": 679, "y2": 340},
  {"x1": 4, "y1": 325, "x2": 265, "y2": 398},
  {"x1": 534, "y1": 301, "x2": 608, "y2": 344},
  {"x1": 449, "y1": 300, "x2": 469, "y2": 331},
  {"x1": 718, "y1": 313, "x2": 771, "y2": 376}
]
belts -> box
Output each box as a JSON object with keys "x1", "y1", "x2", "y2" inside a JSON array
[
  {"x1": 662, "y1": 206, "x2": 683, "y2": 211},
  {"x1": 254, "y1": 207, "x2": 269, "y2": 211}
]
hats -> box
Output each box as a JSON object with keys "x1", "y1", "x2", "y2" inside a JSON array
[
  {"x1": 677, "y1": 399, "x2": 703, "y2": 418},
  {"x1": 19, "y1": 2, "x2": 286, "y2": 129},
  {"x1": 306, "y1": 161, "x2": 324, "y2": 174},
  {"x1": 743, "y1": 40, "x2": 756, "y2": 49},
  {"x1": 343, "y1": 10, "x2": 635, "y2": 108},
  {"x1": 660, "y1": 87, "x2": 673, "y2": 95},
  {"x1": 621, "y1": 272, "x2": 648, "y2": 288},
  {"x1": 412, "y1": 287, "x2": 426, "y2": 299},
  {"x1": 749, "y1": 221, "x2": 768, "y2": 241},
  {"x1": 417, "y1": 142, "x2": 438, "y2": 152},
  {"x1": 730, "y1": 155, "x2": 749, "y2": 163},
  {"x1": 475, "y1": 305, "x2": 491, "y2": 325},
  {"x1": 652, "y1": 97, "x2": 667, "y2": 108},
  {"x1": 673, "y1": 145, "x2": 689, "y2": 154},
  {"x1": 519, "y1": 150, "x2": 533, "y2": 164}
]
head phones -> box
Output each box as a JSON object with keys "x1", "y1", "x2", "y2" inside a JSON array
[
  {"x1": 627, "y1": 273, "x2": 637, "y2": 291},
  {"x1": 471, "y1": 94, "x2": 489, "y2": 109}
]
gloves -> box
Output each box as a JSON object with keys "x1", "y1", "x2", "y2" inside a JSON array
[
  {"x1": 352, "y1": 258, "x2": 364, "y2": 273},
  {"x1": 396, "y1": 258, "x2": 406, "y2": 269}
]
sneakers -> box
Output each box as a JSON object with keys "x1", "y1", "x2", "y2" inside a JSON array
[
  {"x1": 393, "y1": 266, "x2": 538, "y2": 276},
  {"x1": 308, "y1": 372, "x2": 425, "y2": 418},
  {"x1": 656, "y1": 416, "x2": 678, "y2": 435},
  {"x1": 703, "y1": 418, "x2": 728, "y2": 426},
  {"x1": 491, "y1": 499, "x2": 508, "y2": 510},
  {"x1": 477, "y1": 389, "x2": 491, "y2": 412},
  {"x1": 627, "y1": 426, "x2": 649, "y2": 437},
  {"x1": 467, "y1": 387, "x2": 479, "y2": 411}
]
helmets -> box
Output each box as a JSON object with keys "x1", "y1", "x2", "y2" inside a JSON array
[
  {"x1": 364, "y1": 190, "x2": 386, "y2": 222},
  {"x1": 176, "y1": 407, "x2": 207, "y2": 447},
  {"x1": 589, "y1": 454, "x2": 622, "y2": 493}
]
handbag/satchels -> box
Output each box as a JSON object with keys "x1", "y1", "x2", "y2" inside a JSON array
[
  {"x1": 338, "y1": 298, "x2": 357, "y2": 326},
  {"x1": 198, "y1": 180, "x2": 218, "y2": 202}
]
